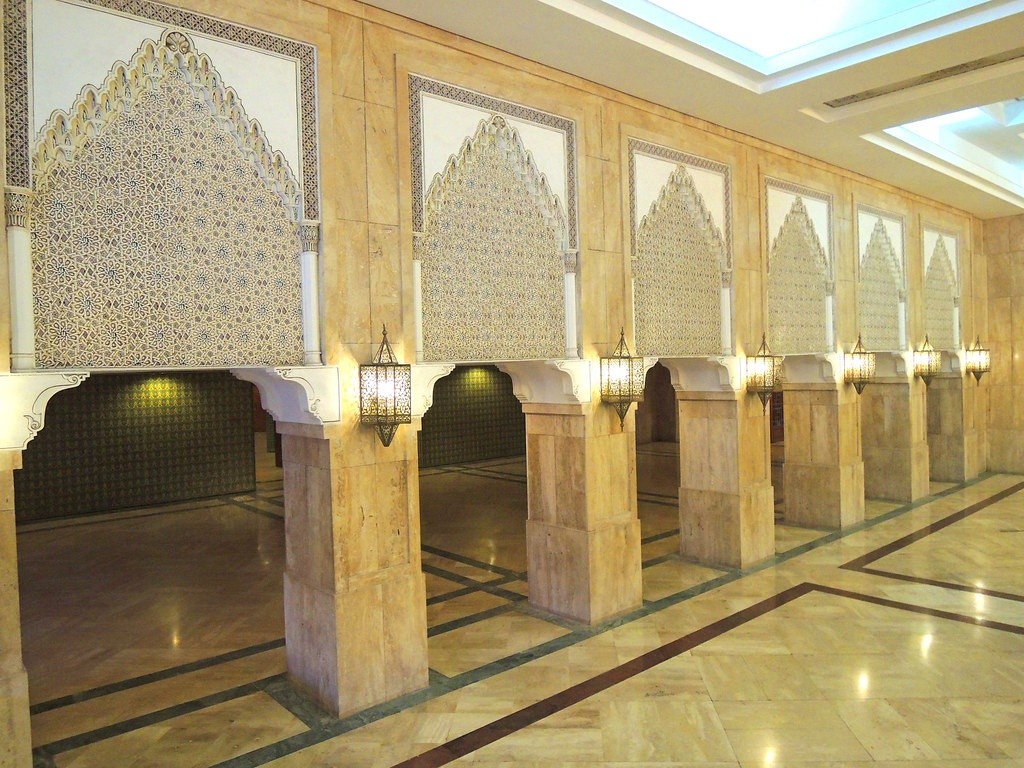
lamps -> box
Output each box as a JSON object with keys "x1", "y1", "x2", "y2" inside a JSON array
[
  {"x1": 844, "y1": 332, "x2": 876, "y2": 394},
  {"x1": 965, "y1": 335, "x2": 990, "y2": 386},
  {"x1": 913, "y1": 333, "x2": 942, "y2": 392},
  {"x1": 359, "y1": 324, "x2": 412, "y2": 447},
  {"x1": 600, "y1": 326, "x2": 645, "y2": 431},
  {"x1": 746, "y1": 332, "x2": 783, "y2": 418}
]
